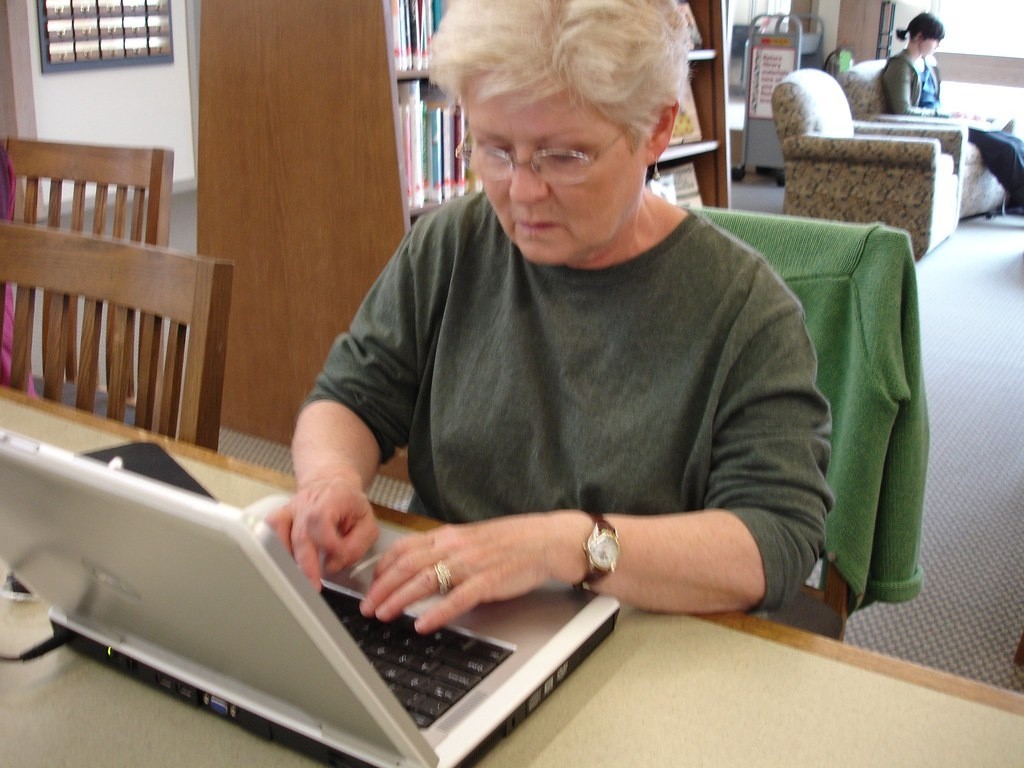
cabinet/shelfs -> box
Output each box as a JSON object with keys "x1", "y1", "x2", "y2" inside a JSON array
[
  {"x1": 730, "y1": 11, "x2": 827, "y2": 188},
  {"x1": 198, "y1": 0, "x2": 733, "y2": 482}
]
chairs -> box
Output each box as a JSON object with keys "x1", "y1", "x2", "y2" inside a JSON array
[
  {"x1": 693, "y1": 202, "x2": 910, "y2": 634},
  {"x1": 0, "y1": 136, "x2": 236, "y2": 449}
]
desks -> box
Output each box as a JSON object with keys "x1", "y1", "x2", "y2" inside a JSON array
[{"x1": 0, "y1": 388, "x2": 1024, "y2": 768}]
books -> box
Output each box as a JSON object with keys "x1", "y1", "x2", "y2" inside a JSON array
[
  {"x1": 391, "y1": 0, "x2": 483, "y2": 207},
  {"x1": 645, "y1": 1, "x2": 705, "y2": 206}
]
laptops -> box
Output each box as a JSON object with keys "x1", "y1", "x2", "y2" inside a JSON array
[{"x1": 0, "y1": 428, "x2": 621, "y2": 768}]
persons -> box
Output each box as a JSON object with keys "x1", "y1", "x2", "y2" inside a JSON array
[
  {"x1": 260, "y1": 0, "x2": 833, "y2": 632},
  {"x1": 881, "y1": 12, "x2": 1024, "y2": 210}
]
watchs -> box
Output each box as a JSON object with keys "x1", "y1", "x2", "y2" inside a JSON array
[{"x1": 583, "y1": 511, "x2": 620, "y2": 585}]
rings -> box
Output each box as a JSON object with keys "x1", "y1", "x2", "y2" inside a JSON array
[{"x1": 434, "y1": 560, "x2": 453, "y2": 595}]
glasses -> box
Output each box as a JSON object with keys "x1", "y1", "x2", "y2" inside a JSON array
[{"x1": 453, "y1": 126, "x2": 626, "y2": 186}]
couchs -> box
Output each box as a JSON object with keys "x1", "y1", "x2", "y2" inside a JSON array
[
  {"x1": 774, "y1": 68, "x2": 970, "y2": 258},
  {"x1": 844, "y1": 56, "x2": 1014, "y2": 218}
]
